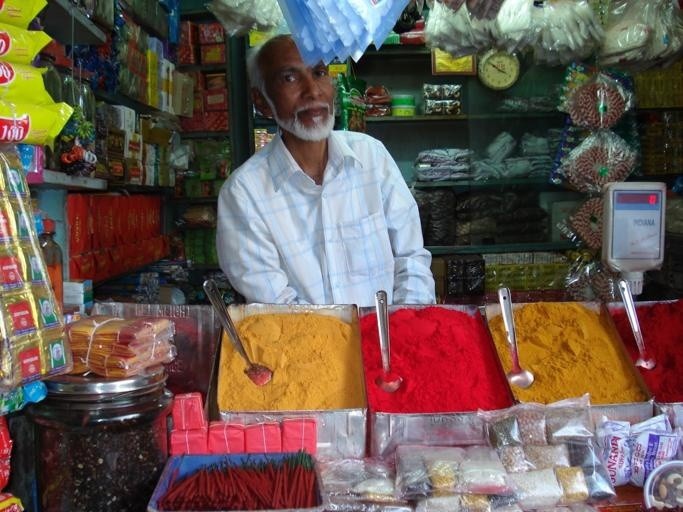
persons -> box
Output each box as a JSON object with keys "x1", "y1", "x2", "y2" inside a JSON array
[{"x1": 213, "y1": 32, "x2": 437, "y2": 305}]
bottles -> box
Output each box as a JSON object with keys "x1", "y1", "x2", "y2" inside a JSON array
[
  {"x1": 38, "y1": 219, "x2": 64, "y2": 316},
  {"x1": 34, "y1": 39, "x2": 96, "y2": 126}
]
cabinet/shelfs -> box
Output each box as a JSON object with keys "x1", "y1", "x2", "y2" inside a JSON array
[
  {"x1": 244, "y1": 41, "x2": 683, "y2": 267},
  {"x1": 0, "y1": 1, "x2": 243, "y2": 302}
]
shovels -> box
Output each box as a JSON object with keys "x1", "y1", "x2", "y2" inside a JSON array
[
  {"x1": 375, "y1": 290, "x2": 403, "y2": 393},
  {"x1": 497, "y1": 287, "x2": 534, "y2": 389},
  {"x1": 617, "y1": 277, "x2": 656, "y2": 370},
  {"x1": 202, "y1": 278, "x2": 273, "y2": 386}
]
